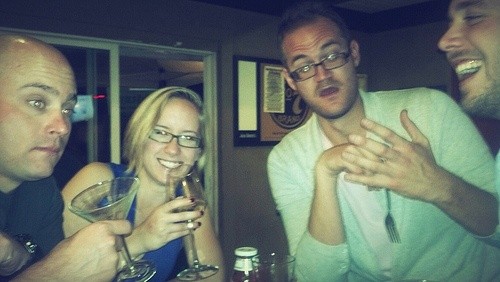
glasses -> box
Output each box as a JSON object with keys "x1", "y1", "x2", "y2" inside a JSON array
[
  {"x1": 148, "y1": 129, "x2": 203, "y2": 149},
  {"x1": 290, "y1": 49, "x2": 350, "y2": 83}
]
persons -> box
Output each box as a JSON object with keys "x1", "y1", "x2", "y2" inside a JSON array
[
  {"x1": 438, "y1": 1, "x2": 500, "y2": 122},
  {"x1": 58, "y1": 86, "x2": 226, "y2": 281},
  {"x1": 0, "y1": 31, "x2": 132, "y2": 282},
  {"x1": 265, "y1": 6, "x2": 499, "y2": 282}
]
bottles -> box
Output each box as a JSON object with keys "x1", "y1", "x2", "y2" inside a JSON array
[{"x1": 231, "y1": 246, "x2": 258, "y2": 282}]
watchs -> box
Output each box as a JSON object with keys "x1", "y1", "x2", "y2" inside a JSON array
[{"x1": 2, "y1": 233, "x2": 45, "y2": 279}]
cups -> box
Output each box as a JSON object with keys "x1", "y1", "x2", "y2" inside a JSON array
[{"x1": 251, "y1": 253, "x2": 296, "y2": 282}]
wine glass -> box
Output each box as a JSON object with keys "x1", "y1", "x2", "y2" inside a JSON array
[
  {"x1": 68, "y1": 177, "x2": 156, "y2": 282},
  {"x1": 167, "y1": 164, "x2": 219, "y2": 281}
]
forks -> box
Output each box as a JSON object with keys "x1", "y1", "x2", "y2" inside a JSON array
[{"x1": 385, "y1": 189, "x2": 402, "y2": 244}]
